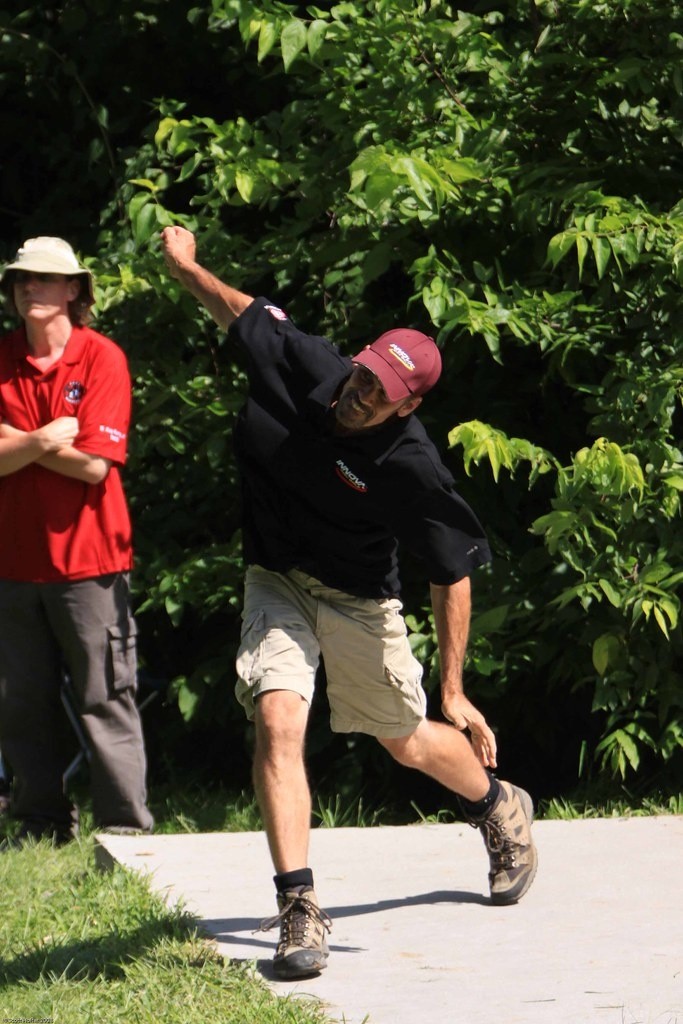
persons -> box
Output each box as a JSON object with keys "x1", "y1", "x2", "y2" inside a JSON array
[
  {"x1": 0, "y1": 233, "x2": 160, "y2": 847},
  {"x1": 158, "y1": 224, "x2": 539, "y2": 979}
]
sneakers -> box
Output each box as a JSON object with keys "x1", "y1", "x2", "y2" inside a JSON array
[
  {"x1": 457, "y1": 779, "x2": 538, "y2": 905},
  {"x1": 252, "y1": 885, "x2": 332, "y2": 979}
]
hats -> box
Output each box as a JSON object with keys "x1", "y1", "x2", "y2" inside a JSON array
[
  {"x1": 0, "y1": 236, "x2": 95, "y2": 326},
  {"x1": 351, "y1": 328, "x2": 442, "y2": 402}
]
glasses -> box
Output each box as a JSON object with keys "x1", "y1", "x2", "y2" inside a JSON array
[{"x1": 15, "y1": 273, "x2": 60, "y2": 283}]
